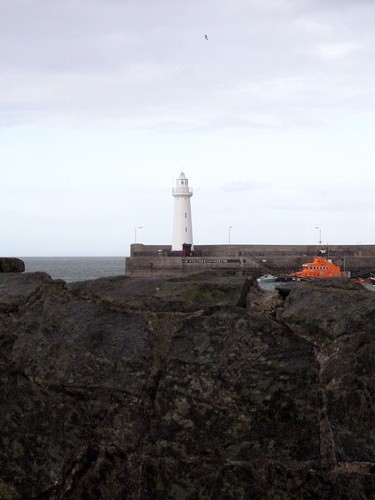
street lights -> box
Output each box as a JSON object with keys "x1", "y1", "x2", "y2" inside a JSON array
[
  {"x1": 229, "y1": 226, "x2": 232, "y2": 245},
  {"x1": 314, "y1": 227, "x2": 322, "y2": 245},
  {"x1": 134, "y1": 226, "x2": 142, "y2": 243}
]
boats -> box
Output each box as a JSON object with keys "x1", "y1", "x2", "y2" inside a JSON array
[{"x1": 255, "y1": 258, "x2": 369, "y2": 294}]
